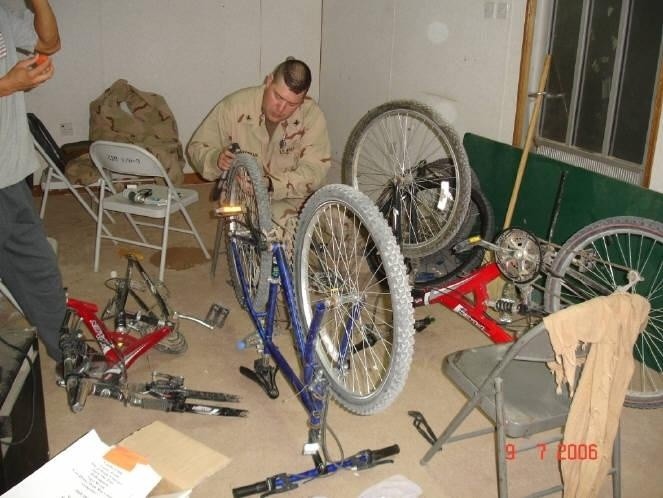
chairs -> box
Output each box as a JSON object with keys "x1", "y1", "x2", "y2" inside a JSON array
[
  {"x1": 89, "y1": 140, "x2": 212, "y2": 287},
  {"x1": 27, "y1": 113, "x2": 119, "y2": 246},
  {"x1": 420, "y1": 293, "x2": 641, "y2": 498}
]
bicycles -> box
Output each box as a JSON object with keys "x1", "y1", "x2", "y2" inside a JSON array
[
  {"x1": 210, "y1": 153, "x2": 414, "y2": 498},
  {"x1": 341, "y1": 101, "x2": 663, "y2": 410},
  {"x1": 55, "y1": 245, "x2": 249, "y2": 419}
]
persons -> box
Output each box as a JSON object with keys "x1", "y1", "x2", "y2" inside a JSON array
[
  {"x1": 0, "y1": 0, "x2": 127, "y2": 386},
  {"x1": 187, "y1": 60, "x2": 331, "y2": 308}
]
